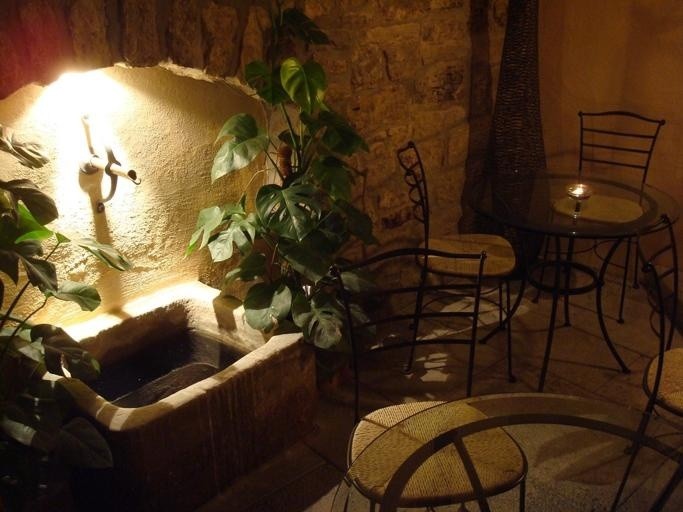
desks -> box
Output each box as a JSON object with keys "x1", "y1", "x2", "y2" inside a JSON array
[
  {"x1": 333, "y1": 393, "x2": 683, "y2": 512},
  {"x1": 464, "y1": 165, "x2": 681, "y2": 392}
]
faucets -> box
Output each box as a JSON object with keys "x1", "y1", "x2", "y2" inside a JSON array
[{"x1": 80, "y1": 152, "x2": 142, "y2": 186}]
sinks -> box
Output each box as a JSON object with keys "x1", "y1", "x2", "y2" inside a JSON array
[{"x1": 47, "y1": 279, "x2": 317, "y2": 511}]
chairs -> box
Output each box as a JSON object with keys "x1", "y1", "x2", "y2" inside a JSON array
[
  {"x1": 334, "y1": 247, "x2": 528, "y2": 511},
  {"x1": 531, "y1": 112, "x2": 668, "y2": 323},
  {"x1": 612, "y1": 215, "x2": 683, "y2": 511},
  {"x1": 398, "y1": 140, "x2": 520, "y2": 396}
]
